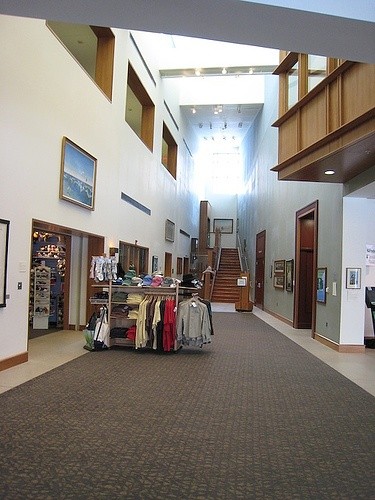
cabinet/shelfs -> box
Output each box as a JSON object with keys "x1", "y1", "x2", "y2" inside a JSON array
[
  {"x1": 29, "y1": 239, "x2": 66, "y2": 329},
  {"x1": 90, "y1": 282, "x2": 199, "y2": 351}
]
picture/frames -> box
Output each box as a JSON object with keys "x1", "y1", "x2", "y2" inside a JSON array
[
  {"x1": 346, "y1": 267, "x2": 361, "y2": 289},
  {"x1": 274, "y1": 275, "x2": 285, "y2": 289},
  {"x1": 165, "y1": 219, "x2": 176, "y2": 242},
  {"x1": 274, "y1": 260, "x2": 285, "y2": 273},
  {"x1": 58, "y1": 136, "x2": 97, "y2": 211},
  {"x1": 0, "y1": 218, "x2": 9, "y2": 307},
  {"x1": 286, "y1": 258, "x2": 294, "y2": 292},
  {"x1": 213, "y1": 218, "x2": 233, "y2": 234},
  {"x1": 316, "y1": 268, "x2": 327, "y2": 303}
]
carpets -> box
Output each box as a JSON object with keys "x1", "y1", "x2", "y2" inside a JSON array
[{"x1": 0, "y1": 313, "x2": 374, "y2": 500}]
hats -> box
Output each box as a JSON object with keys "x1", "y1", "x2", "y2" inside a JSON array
[{"x1": 122, "y1": 269, "x2": 173, "y2": 287}]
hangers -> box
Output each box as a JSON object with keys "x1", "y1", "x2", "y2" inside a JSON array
[
  {"x1": 145, "y1": 292, "x2": 176, "y2": 300},
  {"x1": 187, "y1": 295, "x2": 200, "y2": 303}
]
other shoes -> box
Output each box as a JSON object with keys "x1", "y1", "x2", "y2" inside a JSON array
[{"x1": 28, "y1": 243, "x2": 66, "y2": 325}]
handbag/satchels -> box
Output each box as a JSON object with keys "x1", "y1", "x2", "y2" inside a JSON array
[{"x1": 92, "y1": 306, "x2": 109, "y2": 350}]
